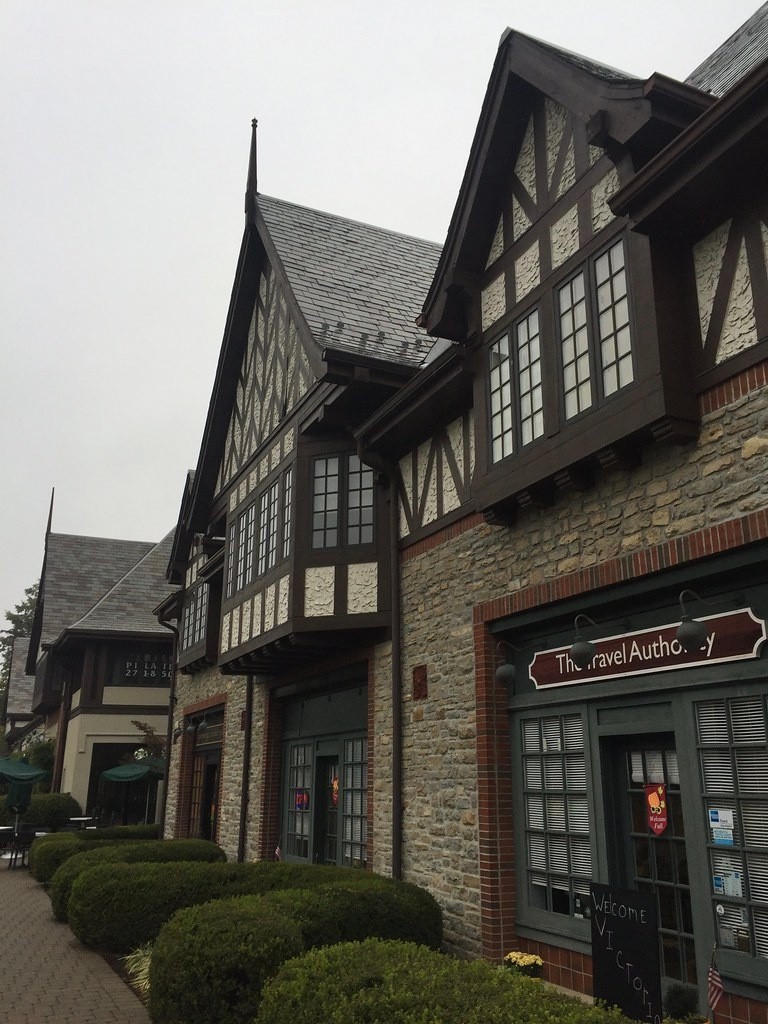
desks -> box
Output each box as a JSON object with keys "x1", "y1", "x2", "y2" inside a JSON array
[
  {"x1": 35, "y1": 832, "x2": 47, "y2": 837},
  {"x1": 70, "y1": 816, "x2": 98, "y2": 830},
  {"x1": 0, "y1": 826, "x2": 14, "y2": 855}
]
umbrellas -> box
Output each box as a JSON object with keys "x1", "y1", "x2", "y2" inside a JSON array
[
  {"x1": 0, "y1": 756, "x2": 52, "y2": 834},
  {"x1": 101, "y1": 762, "x2": 171, "y2": 826}
]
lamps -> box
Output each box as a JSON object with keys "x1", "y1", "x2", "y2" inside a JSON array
[
  {"x1": 173, "y1": 719, "x2": 191, "y2": 740},
  {"x1": 494, "y1": 640, "x2": 547, "y2": 686},
  {"x1": 677, "y1": 589, "x2": 745, "y2": 653},
  {"x1": 571, "y1": 614, "x2": 631, "y2": 668},
  {"x1": 198, "y1": 712, "x2": 215, "y2": 730},
  {"x1": 187, "y1": 715, "x2": 202, "y2": 733}
]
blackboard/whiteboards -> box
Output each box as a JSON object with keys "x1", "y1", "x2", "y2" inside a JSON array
[{"x1": 589, "y1": 882, "x2": 663, "y2": 1023}]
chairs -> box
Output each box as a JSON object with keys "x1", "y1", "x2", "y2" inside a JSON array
[{"x1": 1, "y1": 828, "x2": 34, "y2": 871}]
